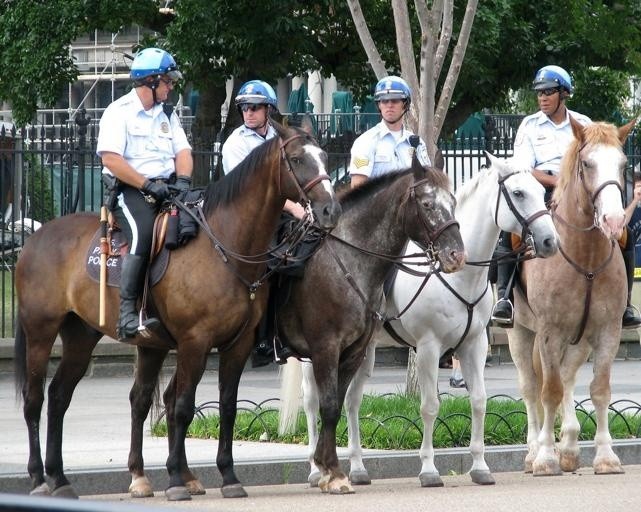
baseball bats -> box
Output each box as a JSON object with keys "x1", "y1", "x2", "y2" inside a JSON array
[{"x1": 102, "y1": 174, "x2": 121, "y2": 209}]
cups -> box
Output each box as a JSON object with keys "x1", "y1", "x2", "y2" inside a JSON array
[
  {"x1": 240, "y1": 103, "x2": 267, "y2": 112},
  {"x1": 153, "y1": 76, "x2": 176, "y2": 87},
  {"x1": 535, "y1": 88, "x2": 564, "y2": 98}
]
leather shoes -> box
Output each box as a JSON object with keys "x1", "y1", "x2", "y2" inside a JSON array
[{"x1": 144, "y1": 175, "x2": 192, "y2": 205}]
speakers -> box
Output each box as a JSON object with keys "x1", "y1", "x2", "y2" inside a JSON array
[
  {"x1": 493, "y1": 230, "x2": 521, "y2": 328},
  {"x1": 251, "y1": 279, "x2": 293, "y2": 367},
  {"x1": 622, "y1": 247, "x2": 641, "y2": 326},
  {"x1": 116, "y1": 253, "x2": 161, "y2": 342}
]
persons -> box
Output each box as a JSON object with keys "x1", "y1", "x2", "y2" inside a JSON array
[
  {"x1": 493, "y1": 65, "x2": 641, "y2": 329},
  {"x1": 348, "y1": 76, "x2": 431, "y2": 299},
  {"x1": 95, "y1": 46, "x2": 194, "y2": 342},
  {"x1": 625, "y1": 172, "x2": 641, "y2": 267},
  {"x1": 221, "y1": 80, "x2": 315, "y2": 368}
]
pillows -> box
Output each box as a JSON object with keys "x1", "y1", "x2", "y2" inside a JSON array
[
  {"x1": 130, "y1": 48, "x2": 184, "y2": 82},
  {"x1": 374, "y1": 75, "x2": 411, "y2": 105},
  {"x1": 530, "y1": 65, "x2": 572, "y2": 93},
  {"x1": 235, "y1": 79, "x2": 278, "y2": 111}
]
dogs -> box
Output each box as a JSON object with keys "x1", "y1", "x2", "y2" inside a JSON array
[
  {"x1": 276, "y1": 134, "x2": 563, "y2": 489},
  {"x1": 505, "y1": 111, "x2": 639, "y2": 477},
  {"x1": 126, "y1": 147, "x2": 471, "y2": 500},
  {"x1": 12, "y1": 111, "x2": 345, "y2": 503}
]
